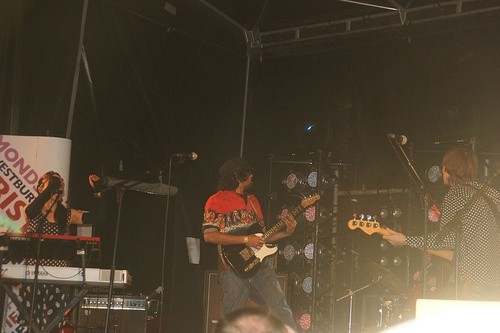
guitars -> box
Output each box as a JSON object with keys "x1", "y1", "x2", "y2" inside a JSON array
[
  {"x1": 347, "y1": 214, "x2": 459, "y2": 268},
  {"x1": 218, "y1": 193, "x2": 321, "y2": 280}
]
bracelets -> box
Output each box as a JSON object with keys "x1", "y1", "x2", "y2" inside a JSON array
[{"x1": 243, "y1": 235, "x2": 248, "y2": 245}]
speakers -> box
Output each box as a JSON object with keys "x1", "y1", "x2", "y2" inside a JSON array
[
  {"x1": 202, "y1": 270, "x2": 288, "y2": 333},
  {"x1": 76, "y1": 309, "x2": 148, "y2": 333}
]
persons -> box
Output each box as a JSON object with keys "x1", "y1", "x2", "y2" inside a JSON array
[
  {"x1": 18, "y1": 171, "x2": 106, "y2": 333},
  {"x1": 214, "y1": 306, "x2": 289, "y2": 333},
  {"x1": 382, "y1": 146, "x2": 500, "y2": 302},
  {"x1": 384, "y1": 271, "x2": 440, "y2": 325},
  {"x1": 202, "y1": 157, "x2": 298, "y2": 333}
]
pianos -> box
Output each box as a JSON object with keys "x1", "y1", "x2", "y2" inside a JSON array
[{"x1": 0, "y1": 230, "x2": 134, "y2": 333}]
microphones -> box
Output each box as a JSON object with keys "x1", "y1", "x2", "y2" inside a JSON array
[
  {"x1": 388, "y1": 134, "x2": 408, "y2": 145},
  {"x1": 172, "y1": 151, "x2": 198, "y2": 160}
]
